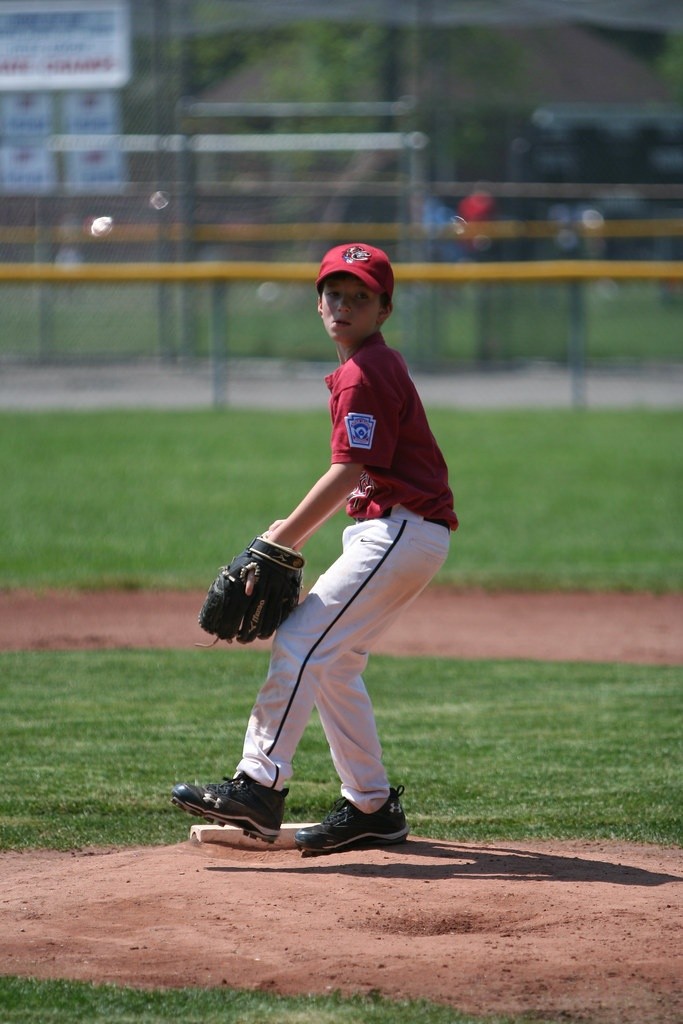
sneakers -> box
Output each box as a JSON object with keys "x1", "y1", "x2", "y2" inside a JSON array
[
  {"x1": 170, "y1": 771, "x2": 289, "y2": 844},
  {"x1": 294, "y1": 785, "x2": 410, "y2": 852}
]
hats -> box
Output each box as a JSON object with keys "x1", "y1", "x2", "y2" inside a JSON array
[{"x1": 315, "y1": 242, "x2": 395, "y2": 303}]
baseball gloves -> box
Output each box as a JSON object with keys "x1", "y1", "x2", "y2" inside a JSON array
[{"x1": 199, "y1": 539, "x2": 303, "y2": 646}]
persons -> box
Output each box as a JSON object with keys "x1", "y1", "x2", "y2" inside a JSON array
[
  {"x1": 410, "y1": 187, "x2": 499, "y2": 260},
  {"x1": 170, "y1": 243, "x2": 458, "y2": 853}
]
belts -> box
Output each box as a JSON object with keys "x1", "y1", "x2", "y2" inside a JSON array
[{"x1": 380, "y1": 506, "x2": 448, "y2": 529}]
